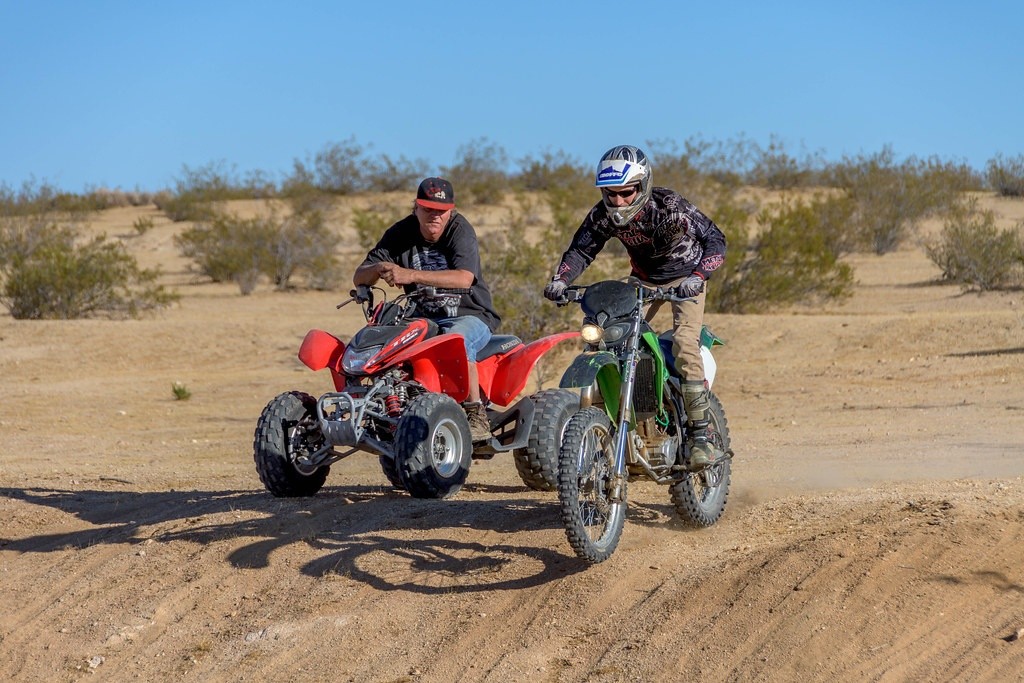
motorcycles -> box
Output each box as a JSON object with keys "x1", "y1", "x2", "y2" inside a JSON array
[
  {"x1": 252, "y1": 283, "x2": 597, "y2": 500},
  {"x1": 543, "y1": 276, "x2": 734, "y2": 566}
]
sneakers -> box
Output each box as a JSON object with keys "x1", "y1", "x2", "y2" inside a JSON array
[{"x1": 463, "y1": 401, "x2": 493, "y2": 441}]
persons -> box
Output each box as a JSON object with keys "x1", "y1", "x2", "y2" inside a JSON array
[
  {"x1": 543, "y1": 145, "x2": 726, "y2": 470},
  {"x1": 353, "y1": 177, "x2": 501, "y2": 443}
]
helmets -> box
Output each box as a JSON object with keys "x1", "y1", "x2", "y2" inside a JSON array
[{"x1": 595, "y1": 145, "x2": 653, "y2": 227}]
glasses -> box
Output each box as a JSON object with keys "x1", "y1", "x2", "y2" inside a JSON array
[{"x1": 603, "y1": 187, "x2": 637, "y2": 198}]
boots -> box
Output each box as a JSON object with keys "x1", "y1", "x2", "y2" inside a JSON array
[{"x1": 678, "y1": 379, "x2": 719, "y2": 465}]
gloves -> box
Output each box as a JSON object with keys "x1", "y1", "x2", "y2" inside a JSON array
[
  {"x1": 677, "y1": 275, "x2": 703, "y2": 298},
  {"x1": 543, "y1": 279, "x2": 567, "y2": 302}
]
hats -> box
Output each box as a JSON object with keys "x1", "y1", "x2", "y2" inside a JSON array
[{"x1": 414, "y1": 176, "x2": 456, "y2": 211}]
